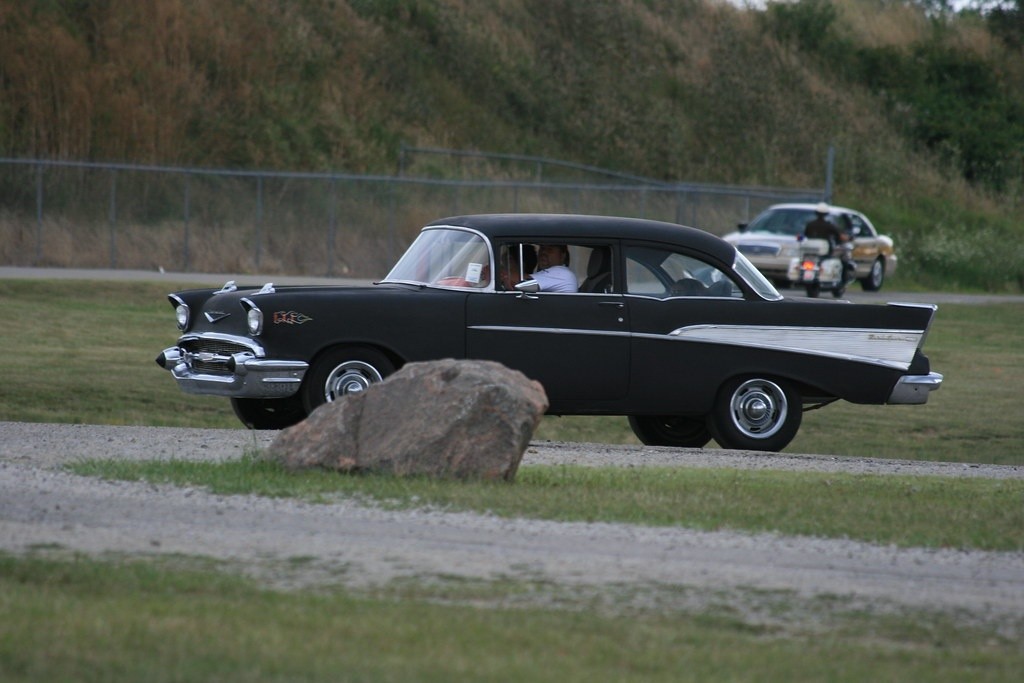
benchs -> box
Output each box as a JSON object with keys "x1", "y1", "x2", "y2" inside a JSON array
[{"x1": 670, "y1": 278, "x2": 732, "y2": 296}]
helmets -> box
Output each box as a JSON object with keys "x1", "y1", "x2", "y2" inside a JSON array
[{"x1": 815, "y1": 202, "x2": 830, "y2": 214}]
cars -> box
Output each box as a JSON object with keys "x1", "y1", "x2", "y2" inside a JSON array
[
  {"x1": 156, "y1": 214, "x2": 945, "y2": 453},
  {"x1": 720, "y1": 200, "x2": 899, "y2": 301}
]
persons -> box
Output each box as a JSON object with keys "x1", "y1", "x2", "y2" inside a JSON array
[
  {"x1": 482, "y1": 243, "x2": 578, "y2": 293},
  {"x1": 803, "y1": 202, "x2": 855, "y2": 287}
]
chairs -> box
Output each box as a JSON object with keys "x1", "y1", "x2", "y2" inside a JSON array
[{"x1": 579, "y1": 247, "x2": 613, "y2": 293}]
